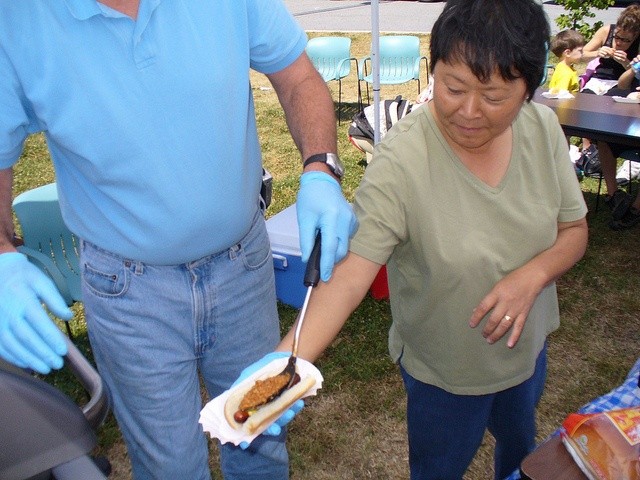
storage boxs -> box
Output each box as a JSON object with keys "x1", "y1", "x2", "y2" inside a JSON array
[{"x1": 264, "y1": 202, "x2": 308, "y2": 307}]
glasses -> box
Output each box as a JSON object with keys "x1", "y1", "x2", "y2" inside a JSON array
[{"x1": 613, "y1": 30, "x2": 634, "y2": 43}]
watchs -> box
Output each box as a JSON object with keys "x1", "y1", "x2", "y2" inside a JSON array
[{"x1": 303, "y1": 153, "x2": 345, "y2": 178}]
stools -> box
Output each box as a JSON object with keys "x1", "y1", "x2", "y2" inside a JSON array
[{"x1": 584, "y1": 139, "x2": 639, "y2": 215}]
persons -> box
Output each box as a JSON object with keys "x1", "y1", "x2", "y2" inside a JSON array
[
  {"x1": 0, "y1": 0, "x2": 357, "y2": 480},
  {"x1": 548, "y1": 30, "x2": 582, "y2": 152},
  {"x1": 598, "y1": 54, "x2": 640, "y2": 232},
  {"x1": 576, "y1": 4, "x2": 640, "y2": 173},
  {"x1": 239, "y1": 0, "x2": 589, "y2": 480}
]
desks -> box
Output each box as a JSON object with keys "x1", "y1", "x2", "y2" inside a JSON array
[
  {"x1": 521, "y1": 357, "x2": 639, "y2": 480},
  {"x1": 531, "y1": 93, "x2": 640, "y2": 156}
]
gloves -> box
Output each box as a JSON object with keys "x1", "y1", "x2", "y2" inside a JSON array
[
  {"x1": 296, "y1": 171, "x2": 358, "y2": 283},
  {"x1": 0, "y1": 252, "x2": 72, "y2": 375},
  {"x1": 229, "y1": 351, "x2": 304, "y2": 451}
]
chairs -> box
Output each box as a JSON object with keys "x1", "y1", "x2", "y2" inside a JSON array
[
  {"x1": 12, "y1": 183, "x2": 83, "y2": 342},
  {"x1": 304, "y1": 36, "x2": 363, "y2": 125},
  {"x1": 358, "y1": 34, "x2": 429, "y2": 116}
]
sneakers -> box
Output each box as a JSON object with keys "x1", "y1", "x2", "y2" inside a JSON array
[
  {"x1": 604, "y1": 190, "x2": 629, "y2": 217},
  {"x1": 611, "y1": 207, "x2": 640, "y2": 230},
  {"x1": 616, "y1": 160, "x2": 640, "y2": 185}
]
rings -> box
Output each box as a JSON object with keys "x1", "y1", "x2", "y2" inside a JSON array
[{"x1": 504, "y1": 315, "x2": 514, "y2": 323}]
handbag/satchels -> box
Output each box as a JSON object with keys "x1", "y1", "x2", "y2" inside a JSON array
[{"x1": 577, "y1": 143, "x2": 601, "y2": 174}]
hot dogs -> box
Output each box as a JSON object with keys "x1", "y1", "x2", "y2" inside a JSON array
[{"x1": 224, "y1": 364, "x2": 316, "y2": 437}]
